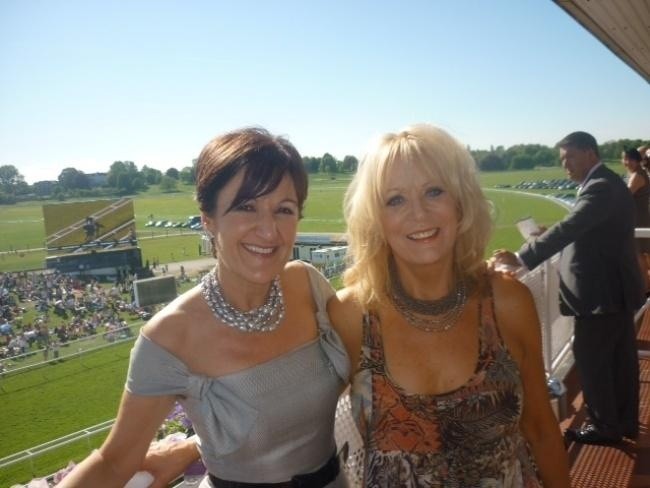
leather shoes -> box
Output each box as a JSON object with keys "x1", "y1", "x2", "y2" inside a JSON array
[{"x1": 564, "y1": 424, "x2": 623, "y2": 445}]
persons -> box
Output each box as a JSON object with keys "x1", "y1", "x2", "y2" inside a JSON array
[
  {"x1": 621, "y1": 145, "x2": 650, "y2": 297},
  {"x1": 84, "y1": 217, "x2": 103, "y2": 241},
  {"x1": 0, "y1": 250, "x2": 199, "y2": 358},
  {"x1": 492, "y1": 131, "x2": 646, "y2": 444},
  {"x1": 48, "y1": 130, "x2": 516, "y2": 488},
  {"x1": 135, "y1": 121, "x2": 572, "y2": 488}
]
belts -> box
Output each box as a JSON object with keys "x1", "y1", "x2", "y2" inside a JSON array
[{"x1": 204, "y1": 442, "x2": 349, "y2": 488}]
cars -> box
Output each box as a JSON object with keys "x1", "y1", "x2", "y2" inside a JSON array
[
  {"x1": 494, "y1": 184, "x2": 510, "y2": 188},
  {"x1": 145, "y1": 216, "x2": 202, "y2": 230},
  {"x1": 557, "y1": 193, "x2": 575, "y2": 199},
  {"x1": 515, "y1": 180, "x2": 580, "y2": 190}
]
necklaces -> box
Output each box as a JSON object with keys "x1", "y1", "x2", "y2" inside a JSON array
[
  {"x1": 202, "y1": 266, "x2": 286, "y2": 332},
  {"x1": 388, "y1": 268, "x2": 472, "y2": 332}
]
